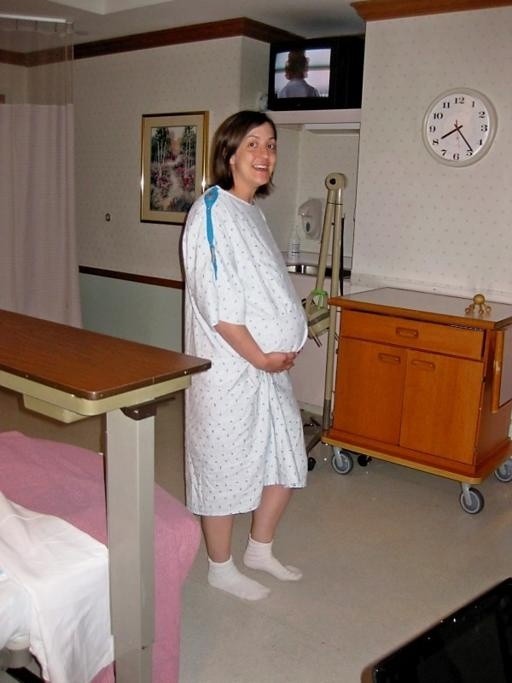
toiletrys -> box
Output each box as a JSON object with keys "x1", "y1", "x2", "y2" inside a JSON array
[{"x1": 287, "y1": 222, "x2": 301, "y2": 256}]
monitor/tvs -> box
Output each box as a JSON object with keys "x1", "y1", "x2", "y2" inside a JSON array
[{"x1": 269, "y1": 33, "x2": 362, "y2": 109}]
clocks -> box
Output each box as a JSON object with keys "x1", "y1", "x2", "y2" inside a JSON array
[{"x1": 420, "y1": 88, "x2": 498, "y2": 168}]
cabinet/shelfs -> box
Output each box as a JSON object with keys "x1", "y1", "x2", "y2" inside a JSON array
[{"x1": 321, "y1": 286, "x2": 511, "y2": 514}]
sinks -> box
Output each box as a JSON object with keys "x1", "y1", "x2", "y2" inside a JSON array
[{"x1": 288, "y1": 262, "x2": 351, "y2": 280}]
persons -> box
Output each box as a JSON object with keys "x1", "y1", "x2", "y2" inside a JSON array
[
  {"x1": 275, "y1": 50, "x2": 321, "y2": 97},
  {"x1": 177, "y1": 107, "x2": 311, "y2": 603}
]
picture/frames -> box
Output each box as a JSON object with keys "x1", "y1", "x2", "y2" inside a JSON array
[{"x1": 141, "y1": 111, "x2": 209, "y2": 226}]
